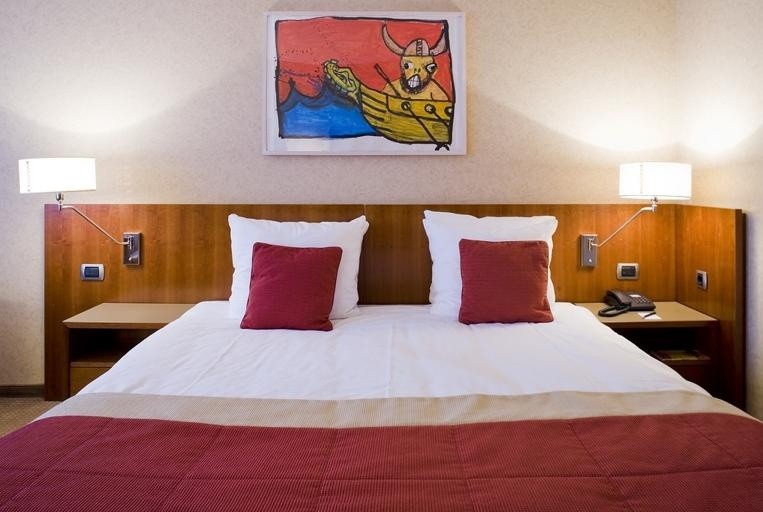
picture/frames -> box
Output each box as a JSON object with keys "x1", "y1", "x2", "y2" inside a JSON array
[{"x1": 262, "y1": 13, "x2": 467, "y2": 157}]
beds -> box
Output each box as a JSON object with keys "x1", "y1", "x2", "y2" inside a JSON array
[{"x1": 1, "y1": 301, "x2": 758, "y2": 511}]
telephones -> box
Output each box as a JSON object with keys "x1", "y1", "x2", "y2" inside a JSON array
[{"x1": 604, "y1": 289, "x2": 656, "y2": 310}]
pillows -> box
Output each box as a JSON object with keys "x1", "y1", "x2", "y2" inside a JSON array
[
  {"x1": 420, "y1": 209, "x2": 558, "y2": 327},
  {"x1": 239, "y1": 239, "x2": 342, "y2": 331},
  {"x1": 225, "y1": 212, "x2": 369, "y2": 331},
  {"x1": 458, "y1": 237, "x2": 555, "y2": 327}
]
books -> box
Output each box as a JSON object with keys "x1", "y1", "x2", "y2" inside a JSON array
[{"x1": 651, "y1": 349, "x2": 711, "y2": 361}]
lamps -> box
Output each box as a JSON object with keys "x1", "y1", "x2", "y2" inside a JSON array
[
  {"x1": 579, "y1": 160, "x2": 690, "y2": 268},
  {"x1": 18, "y1": 156, "x2": 142, "y2": 267}
]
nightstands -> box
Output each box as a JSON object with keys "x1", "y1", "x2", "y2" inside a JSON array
[
  {"x1": 576, "y1": 299, "x2": 717, "y2": 396},
  {"x1": 56, "y1": 302, "x2": 194, "y2": 402}
]
